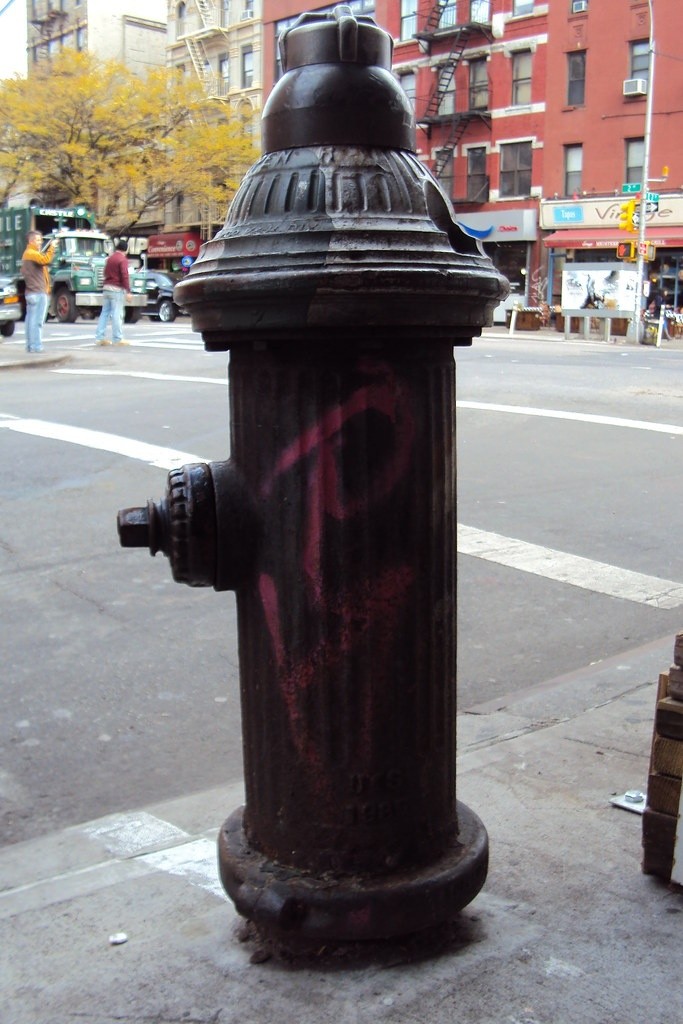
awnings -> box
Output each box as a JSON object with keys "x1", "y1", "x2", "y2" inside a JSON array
[{"x1": 542, "y1": 227, "x2": 682, "y2": 249}]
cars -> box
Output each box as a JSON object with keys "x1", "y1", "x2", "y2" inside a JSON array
[
  {"x1": 140, "y1": 271, "x2": 178, "y2": 324},
  {"x1": 0, "y1": 278, "x2": 23, "y2": 338}
]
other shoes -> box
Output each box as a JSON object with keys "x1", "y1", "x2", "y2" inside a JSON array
[
  {"x1": 96, "y1": 340, "x2": 110, "y2": 346},
  {"x1": 113, "y1": 339, "x2": 129, "y2": 347},
  {"x1": 26, "y1": 347, "x2": 45, "y2": 353}
]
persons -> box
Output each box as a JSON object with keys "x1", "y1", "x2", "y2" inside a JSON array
[
  {"x1": 653, "y1": 286, "x2": 672, "y2": 341},
  {"x1": 21, "y1": 230, "x2": 60, "y2": 352},
  {"x1": 94, "y1": 240, "x2": 134, "y2": 346}
]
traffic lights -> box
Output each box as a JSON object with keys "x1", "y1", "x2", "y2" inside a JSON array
[
  {"x1": 644, "y1": 241, "x2": 655, "y2": 261},
  {"x1": 619, "y1": 200, "x2": 634, "y2": 232},
  {"x1": 616, "y1": 241, "x2": 635, "y2": 259}
]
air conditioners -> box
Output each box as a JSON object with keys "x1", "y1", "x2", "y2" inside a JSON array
[
  {"x1": 622, "y1": 79, "x2": 646, "y2": 97},
  {"x1": 572, "y1": 1, "x2": 588, "y2": 14},
  {"x1": 240, "y1": 10, "x2": 253, "y2": 20}
]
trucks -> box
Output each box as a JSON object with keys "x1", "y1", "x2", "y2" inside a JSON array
[{"x1": 0, "y1": 205, "x2": 149, "y2": 324}]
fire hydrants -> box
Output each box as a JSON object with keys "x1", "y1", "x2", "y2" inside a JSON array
[{"x1": 113, "y1": 4, "x2": 512, "y2": 967}]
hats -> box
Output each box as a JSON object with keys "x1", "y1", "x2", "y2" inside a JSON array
[{"x1": 116, "y1": 240, "x2": 128, "y2": 251}]
